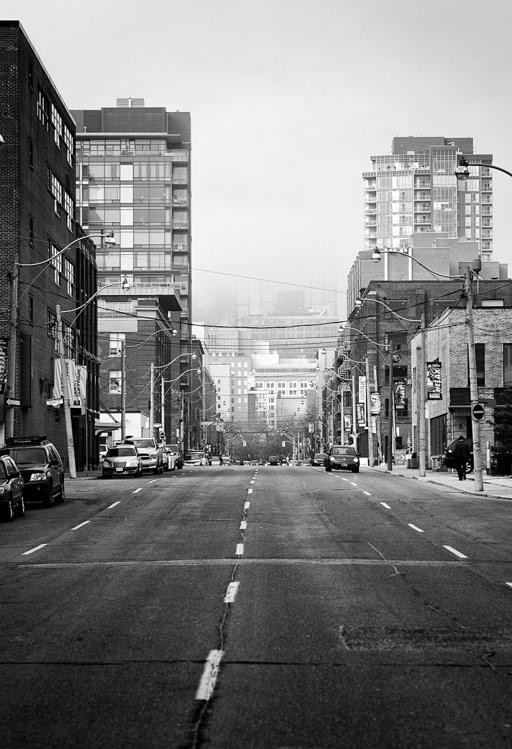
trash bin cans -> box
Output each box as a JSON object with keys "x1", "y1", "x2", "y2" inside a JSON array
[{"x1": 406, "y1": 446, "x2": 418, "y2": 469}]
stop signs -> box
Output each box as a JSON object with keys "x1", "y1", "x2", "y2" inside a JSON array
[{"x1": 471, "y1": 403, "x2": 485, "y2": 421}]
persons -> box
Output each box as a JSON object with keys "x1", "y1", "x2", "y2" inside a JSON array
[
  {"x1": 206, "y1": 451, "x2": 213, "y2": 466},
  {"x1": 218, "y1": 453, "x2": 225, "y2": 466},
  {"x1": 452, "y1": 435, "x2": 470, "y2": 482},
  {"x1": 175, "y1": 454, "x2": 185, "y2": 470}
]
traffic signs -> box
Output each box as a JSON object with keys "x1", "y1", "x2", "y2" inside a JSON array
[{"x1": 6, "y1": 398, "x2": 22, "y2": 407}]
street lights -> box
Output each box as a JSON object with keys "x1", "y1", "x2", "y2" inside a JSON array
[
  {"x1": 53, "y1": 278, "x2": 132, "y2": 479},
  {"x1": 371, "y1": 247, "x2": 485, "y2": 493},
  {"x1": 352, "y1": 297, "x2": 427, "y2": 478},
  {"x1": 453, "y1": 155, "x2": 512, "y2": 182},
  {"x1": 337, "y1": 324, "x2": 394, "y2": 472},
  {"x1": 7, "y1": 231, "x2": 117, "y2": 440},
  {"x1": 120, "y1": 329, "x2": 244, "y2": 452},
  {"x1": 277, "y1": 252, "x2": 374, "y2": 458}
]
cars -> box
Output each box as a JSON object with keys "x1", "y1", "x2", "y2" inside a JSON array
[
  {"x1": 0, "y1": 455, "x2": 27, "y2": 521},
  {"x1": 99, "y1": 437, "x2": 184, "y2": 477},
  {"x1": 325, "y1": 446, "x2": 359, "y2": 473},
  {"x1": 183, "y1": 449, "x2": 327, "y2": 466}
]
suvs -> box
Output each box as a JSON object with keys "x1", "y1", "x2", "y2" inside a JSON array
[{"x1": 0, "y1": 434, "x2": 66, "y2": 505}]
